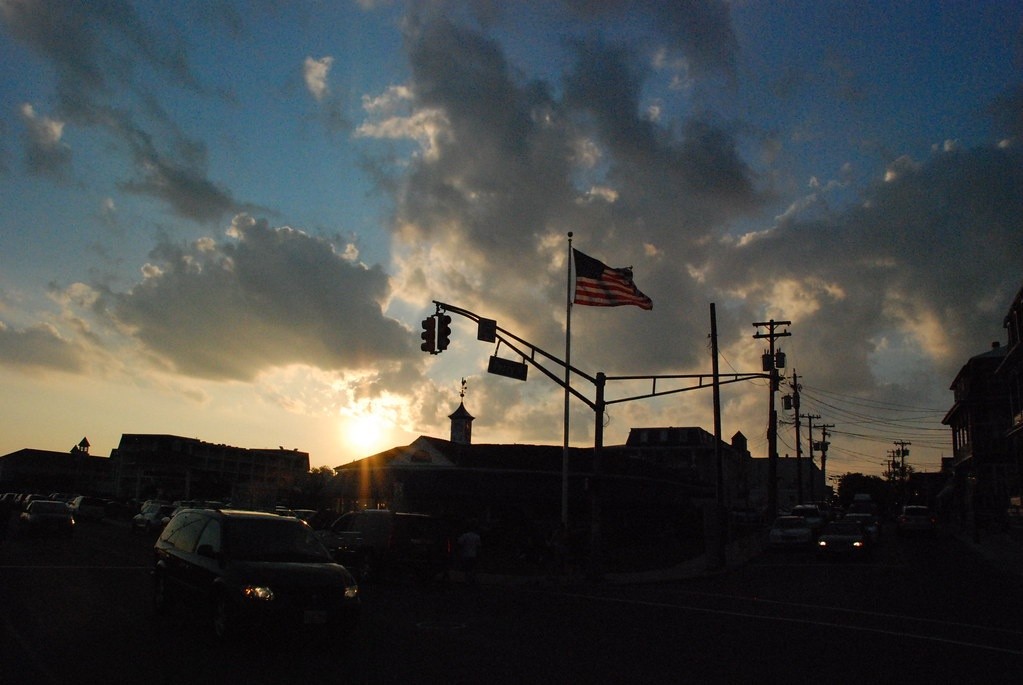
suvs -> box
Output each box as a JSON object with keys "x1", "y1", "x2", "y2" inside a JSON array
[{"x1": 148, "y1": 506, "x2": 361, "y2": 642}]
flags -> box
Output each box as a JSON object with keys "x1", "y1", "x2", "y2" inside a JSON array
[{"x1": 570, "y1": 246, "x2": 654, "y2": 311}]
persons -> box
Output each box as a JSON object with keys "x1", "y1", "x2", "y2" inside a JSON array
[{"x1": 456, "y1": 524, "x2": 482, "y2": 588}]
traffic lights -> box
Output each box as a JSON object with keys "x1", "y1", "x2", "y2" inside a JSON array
[
  {"x1": 440, "y1": 316, "x2": 451, "y2": 348},
  {"x1": 422, "y1": 320, "x2": 428, "y2": 351}
]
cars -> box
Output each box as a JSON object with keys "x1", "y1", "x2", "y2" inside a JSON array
[
  {"x1": 808, "y1": 495, "x2": 833, "y2": 510},
  {"x1": 816, "y1": 519, "x2": 871, "y2": 561},
  {"x1": 66, "y1": 496, "x2": 105, "y2": 523},
  {"x1": 769, "y1": 515, "x2": 814, "y2": 548},
  {"x1": 846, "y1": 513, "x2": 880, "y2": 543},
  {"x1": 319, "y1": 509, "x2": 457, "y2": 595},
  {"x1": 793, "y1": 505, "x2": 823, "y2": 530},
  {"x1": 20, "y1": 500, "x2": 74, "y2": 537},
  {"x1": 848, "y1": 494, "x2": 878, "y2": 518},
  {"x1": 132, "y1": 499, "x2": 225, "y2": 536},
  {"x1": 896, "y1": 505, "x2": 937, "y2": 537},
  {"x1": 803, "y1": 500, "x2": 831, "y2": 520},
  {"x1": 0, "y1": 493, "x2": 77, "y2": 512},
  {"x1": 274, "y1": 507, "x2": 336, "y2": 531}
]
